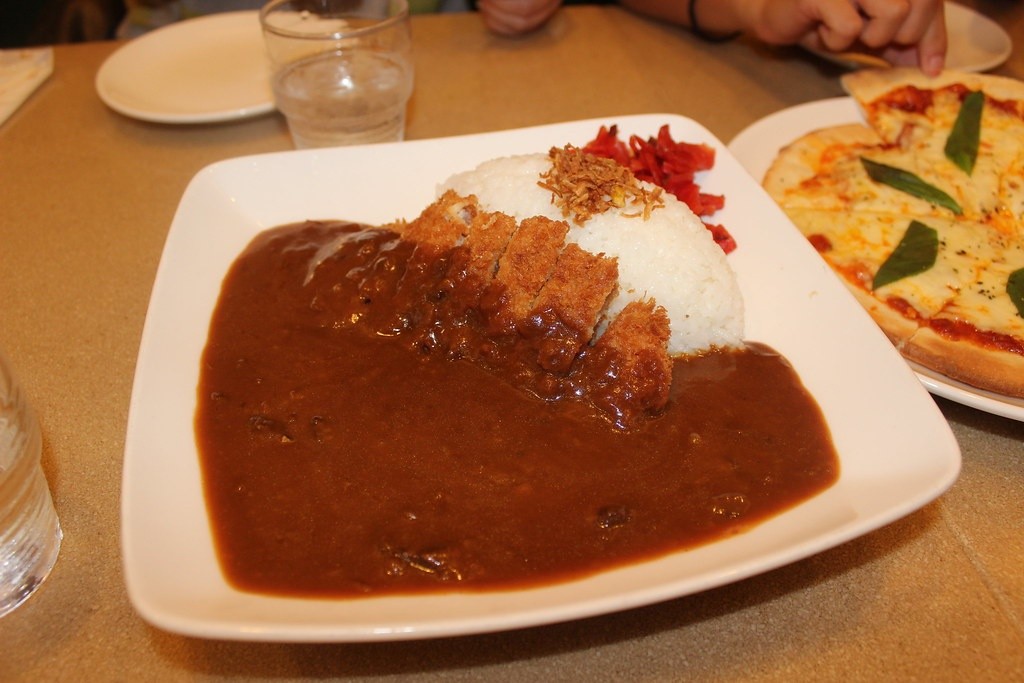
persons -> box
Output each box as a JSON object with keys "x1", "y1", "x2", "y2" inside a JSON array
[
  {"x1": 620, "y1": 0, "x2": 947, "y2": 77},
  {"x1": 476, "y1": 0, "x2": 562, "y2": 36}
]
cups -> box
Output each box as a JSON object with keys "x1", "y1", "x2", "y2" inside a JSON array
[
  {"x1": 1, "y1": 359, "x2": 64, "y2": 619},
  {"x1": 259, "y1": 1, "x2": 412, "y2": 150}
]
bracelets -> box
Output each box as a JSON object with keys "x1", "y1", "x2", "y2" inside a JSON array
[{"x1": 688, "y1": 0, "x2": 743, "y2": 43}]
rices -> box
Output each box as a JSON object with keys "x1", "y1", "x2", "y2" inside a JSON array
[{"x1": 435, "y1": 153, "x2": 745, "y2": 357}]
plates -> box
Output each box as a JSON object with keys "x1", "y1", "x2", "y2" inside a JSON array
[
  {"x1": 123, "y1": 113, "x2": 960, "y2": 643},
  {"x1": 93, "y1": 11, "x2": 358, "y2": 125},
  {"x1": 727, "y1": 97, "x2": 1023, "y2": 428},
  {"x1": 799, "y1": 0, "x2": 1011, "y2": 75}
]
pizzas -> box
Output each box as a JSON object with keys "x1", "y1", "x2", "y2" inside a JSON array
[{"x1": 761, "y1": 66, "x2": 1024, "y2": 401}]
